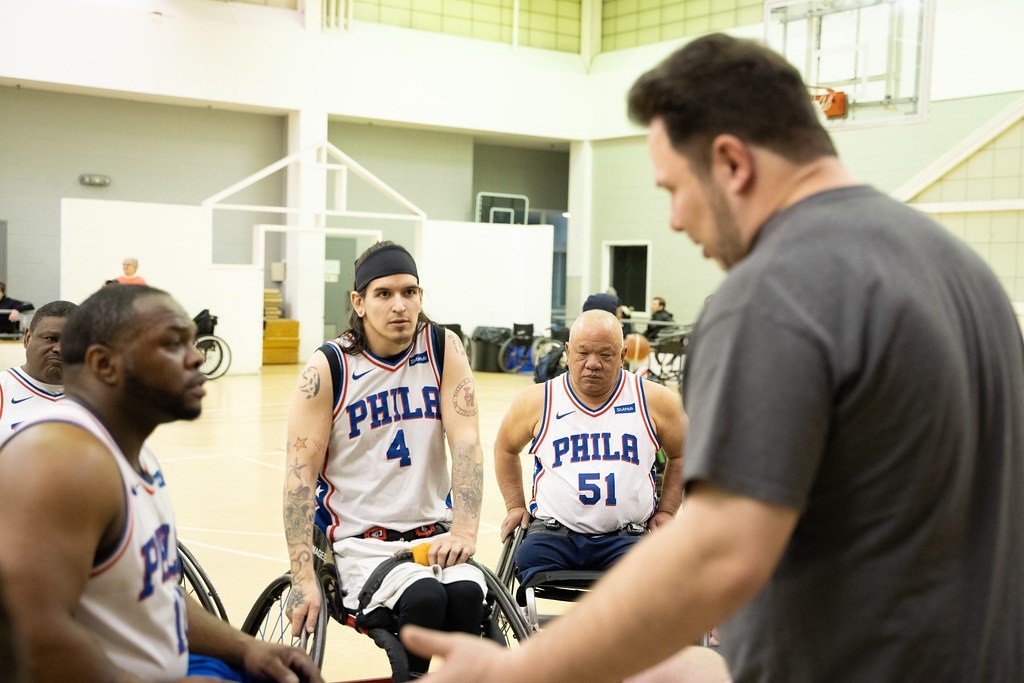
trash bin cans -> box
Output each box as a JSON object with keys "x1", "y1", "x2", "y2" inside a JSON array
[{"x1": 475, "y1": 326, "x2": 511, "y2": 373}]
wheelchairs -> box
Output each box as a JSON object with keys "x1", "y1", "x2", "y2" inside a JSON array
[
  {"x1": 493, "y1": 472, "x2": 710, "y2": 649},
  {"x1": 239, "y1": 524, "x2": 534, "y2": 674},
  {"x1": 498, "y1": 323, "x2": 568, "y2": 374},
  {"x1": 192, "y1": 309, "x2": 232, "y2": 381}
]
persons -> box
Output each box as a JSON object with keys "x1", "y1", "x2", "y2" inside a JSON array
[
  {"x1": 0, "y1": 281, "x2": 35, "y2": 339},
  {"x1": 282, "y1": 240, "x2": 489, "y2": 683},
  {"x1": 642, "y1": 297, "x2": 677, "y2": 341},
  {"x1": 582, "y1": 292, "x2": 623, "y2": 324},
  {"x1": 0, "y1": 301, "x2": 80, "y2": 447},
  {"x1": 114, "y1": 257, "x2": 148, "y2": 287},
  {"x1": 0, "y1": 284, "x2": 325, "y2": 683},
  {"x1": 493, "y1": 310, "x2": 690, "y2": 596},
  {"x1": 399, "y1": 31, "x2": 1024, "y2": 683}
]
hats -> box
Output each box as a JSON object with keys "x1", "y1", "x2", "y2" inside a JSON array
[{"x1": 583, "y1": 294, "x2": 620, "y2": 316}]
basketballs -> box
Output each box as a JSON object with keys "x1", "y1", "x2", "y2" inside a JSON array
[{"x1": 624, "y1": 333, "x2": 651, "y2": 361}]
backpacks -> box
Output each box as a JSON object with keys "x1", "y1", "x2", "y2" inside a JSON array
[{"x1": 534, "y1": 345, "x2": 566, "y2": 383}]
